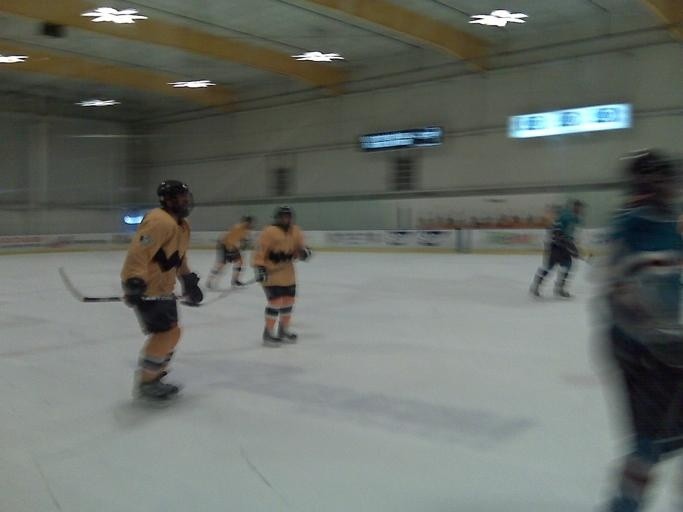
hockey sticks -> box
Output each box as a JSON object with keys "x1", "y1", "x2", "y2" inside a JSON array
[
  {"x1": 180, "y1": 268, "x2": 285, "y2": 307},
  {"x1": 57, "y1": 267, "x2": 189, "y2": 304}
]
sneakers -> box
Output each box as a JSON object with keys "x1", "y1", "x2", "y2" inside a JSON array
[
  {"x1": 278, "y1": 321, "x2": 296, "y2": 340},
  {"x1": 204, "y1": 280, "x2": 213, "y2": 289},
  {"x1": 133, "y1": 371, "x2": 178, "y2": 398},
  {"x1": 528, "y1": 285, "x2": 540, "y2": 297},
  {"x1": 230, "y1": 278, "x2": 242, "y2": 286},
  {"x1": 262, "y1": 327, "x2": 282, "y2": 342}
]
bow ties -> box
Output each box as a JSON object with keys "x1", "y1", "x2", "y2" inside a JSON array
[{"x1": 553, "y1": 287, "x2": 569, "y2": 296}]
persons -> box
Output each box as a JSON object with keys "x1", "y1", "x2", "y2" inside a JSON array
[
  {"x1": 251, "y1": 203, "x2": 314, "y2": 349},
  {"x1": 119, "y1": 178, "x2": 203, "y2": 402},
  {"x1": 595, "y1": 145, "x2": 683, "y2": 512},
  {"x1": 206, "y1": 213, "x2": 255, "y2": 293},
  {"x1": 528, "y1": 197, "x2": 586, "y2": 301},
  {"x1": 416, "y1": 209, "x2": 550, "y2": 229}
]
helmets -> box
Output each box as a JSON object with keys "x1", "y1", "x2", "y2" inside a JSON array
[
  {"x1": 242, "y1": 213, "x2": 253, "y2": 219},
  {"x1": 273, "y1": 203, "x2": 292, "y2": 219},
  {"x1": 156, "y1": 180, "x2": 189, "y2": 196}
]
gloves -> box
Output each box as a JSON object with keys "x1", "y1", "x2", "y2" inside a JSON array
[
  {"x1": 178, "y1": 272, "x2": 203, "y2": 304},
  {"x1": 299, "y1": 248, "x2": 311, "y2": 261},
  {"x1": 123, "y1": 277, "x2": 147, "y2": 308},
  {"x1": 254, "y1": 265, "x2": 267, "y2": 285}
]
